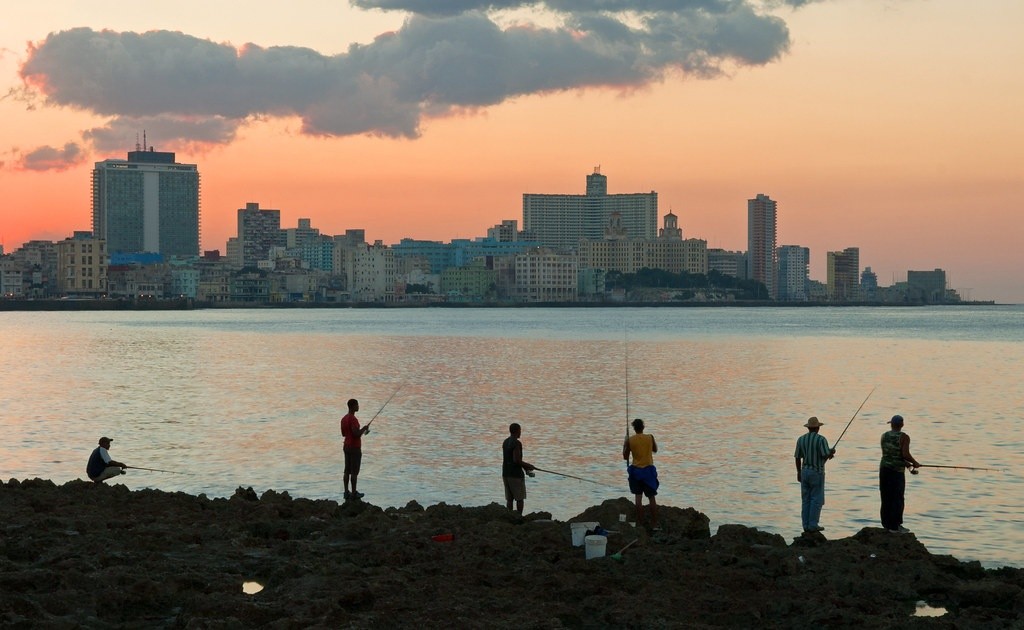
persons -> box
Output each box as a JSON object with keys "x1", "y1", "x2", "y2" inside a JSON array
[
  {"x1": 794, "y1": 417, "x2": 835, "y2": 531},
  {"x1": 341, "y1": 399, "x2": 368, "y2": 499},
  {"x1": 878, "y1": 415, "x2": 920, "y2": 534},
  {"x1": 623, "y1": 419, "x2": 658, "y2": 513},
  {"x1": 86, "y1": 437, "x2": 127, "y2": 484},
  {"x1": 502, "y1": 423, "x2": 534, "y2": 514}
]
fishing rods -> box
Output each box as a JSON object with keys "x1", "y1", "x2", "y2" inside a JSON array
[
  {"x1": 364, "y1": 379, "x2": 409, "y2": 433},
  {"x1": 531, "y1": 464, "x2": 623, "y2": 492},
  {"x1": 831, "y1": 384, "x2": 880, "y2": 454},
  {"x1": 124, "y1": 464, "x2": 197, "y2": 478},
  {"x1": 907, "y1": 462, "x2": 1012, "y2": 481},
  {"x1": 622, "y1": 315, "x2": 634, "y2": 441}
]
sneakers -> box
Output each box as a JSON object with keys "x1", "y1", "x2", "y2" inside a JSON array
[{"x1": 889, "y1": 525, "x2": 910, "y2": 533}]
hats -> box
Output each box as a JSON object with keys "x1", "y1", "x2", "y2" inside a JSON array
[
  {"x1": 887, "y1": 415, "x2": 903, "y2": 425},
  {"x1": 804, "y1": 417, "x2": 824, "y2": 427},
  {"x1": 99, "y1": 437, "x2": 113, "y2": 444}
]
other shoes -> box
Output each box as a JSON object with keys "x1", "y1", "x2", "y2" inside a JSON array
[
  {"x1": 804, "y1": 526, "x2": 824, "y2": 534},
  {"x1": 94, "y1": 481, "x2": 103, "y2": 484},
  {"x1": 344, "y1": 490, "x2": 365, "y2": 500}
]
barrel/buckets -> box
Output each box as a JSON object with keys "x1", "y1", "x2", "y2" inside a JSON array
[
  {"x1": 571, "y1": 522, "x2": 600, "y2": 547},
  {"x1": 584, "y1": 535, "x2": 608, "y2": 560}
]
